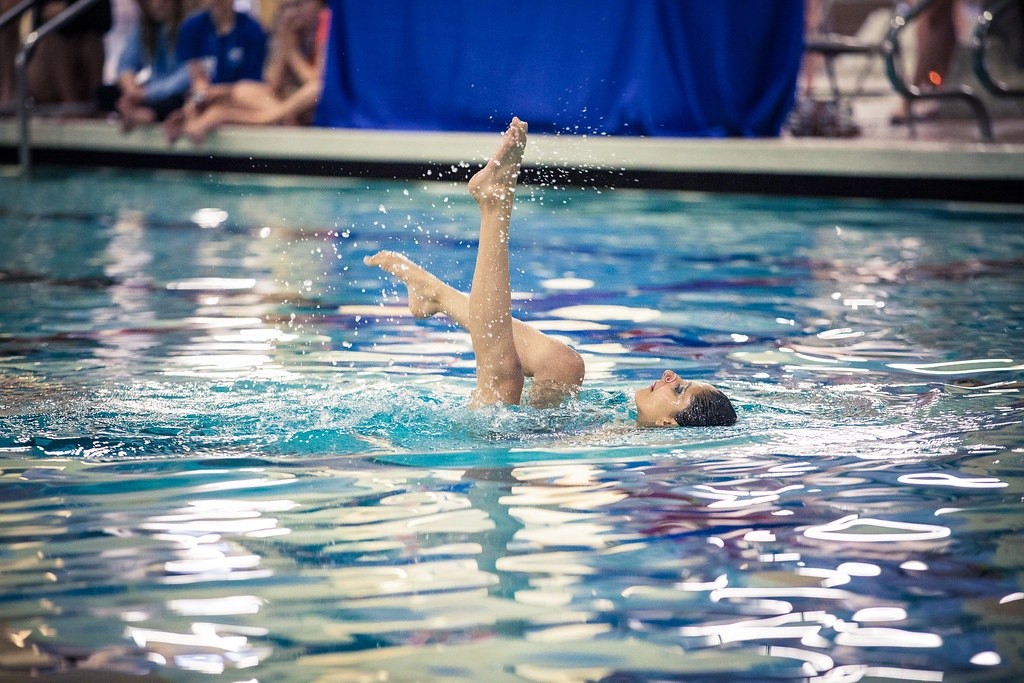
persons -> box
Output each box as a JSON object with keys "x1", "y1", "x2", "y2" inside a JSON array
[
  {"x1": 885, "y1": 0, "x2": 957, "y2": 125},
  {"x1": 0, "y1": 0, "x2": 331, "y2": 144},
  {"x1": 364, "y1": 115, "x2": 738, "y2": 431}
]
